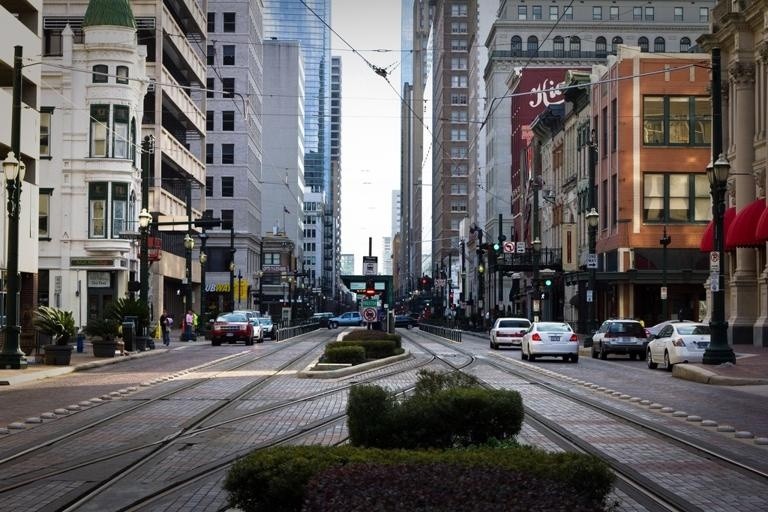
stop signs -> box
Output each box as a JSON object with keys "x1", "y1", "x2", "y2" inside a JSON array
[{"x1": 365, "y1": 287, "x2": 376, "y2": 297}]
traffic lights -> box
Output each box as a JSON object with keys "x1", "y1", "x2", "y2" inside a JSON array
[
  {"x1": 417, "y1": 278, "x2": 422, "y2": 289},
  {"x1": 421, "y1": 278, "x2": 427, "y2": 288},
  {"x1": 492, "y1": 243, "x2": 500, "y2": 252},
  {"x1": 544, "y1": 279, "x2": 552, "y2": 289},
  {"x1": 539, "y1": 292, "x2": 548, "y2": 300},
  {"x1": 427, "y1": 278, "x2": 432, "y2": 287}
]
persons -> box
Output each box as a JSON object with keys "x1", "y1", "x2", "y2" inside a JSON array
[
  {"x1": 185, "y1": 300, "x2": 217, "y2": 340},
  {"x1": 160, "y1": 310, "x2": 169, "y2": 346}
]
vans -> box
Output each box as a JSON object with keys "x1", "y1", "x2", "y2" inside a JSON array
[{"x1": 404, "y1": 313, "x2": 420, "y2": 320}]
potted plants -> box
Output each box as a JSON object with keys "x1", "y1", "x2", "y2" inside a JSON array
[
  {"x1": 104, "y1": 296, "x2": 150, "y2": 352},
  {"x1": 29, "y1": 305, "x2": 74, "y2": 365},
  {"x1": 88, "y1": 316, "x2": 117, "y2": 357}
]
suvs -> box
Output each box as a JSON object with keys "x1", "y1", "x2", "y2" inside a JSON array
[
  {"x1": 488, "y1": 316, "x2": 531, "y2": 351},
  {"x1": 209, "y1": 311, "x2": 255, "y2": 347},
  {"x1": 590, "y1": 319, "x2": 648, "y2": 361}
]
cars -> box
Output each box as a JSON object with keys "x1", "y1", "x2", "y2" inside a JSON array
[
  {"x1": 328, "y1": 312, "x2": 366, "y2": 329},
  {"x1": 645, "y1": 322, "x2": 713, "y2": 372},
  {"x1": 643, "y1": 319, "x2": 695, "y2": 338},
  {"x1": 233, "y1": 309, "x2": 274, "y2": 344},
  {"x1": 394, "y1": 314, "x2": 417, "y2": 330},
  {"x1": 519, "y1": 321, "x2": 579, "y2": 363}
]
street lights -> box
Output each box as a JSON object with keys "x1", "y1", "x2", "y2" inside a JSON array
[
  {"x1": 531, "y1": 235, "x2": 541, "y2": 321},
  {"x1": 224, "y1": 261, "x2": 240, "y2": 313},
  {"x1": 579, "y1": 206, "x2": 599, "y2": 337},
  {"x1": 460, "y1": 270, "x2": 466, "y2": 313},
  {"x1": 257, "y1": 270, "x2": 332, "y2": 327},
  {"x1": 182, "y1": 231, "x2": 198, "y2": 342},
  {"x1": 0, "y1": 151, "x2": 30, "y2": 368},
  {"x1": 131, "y1": 207, "x2": 155, "y2": 350},
  {"x1": 193, "y1": 246, "x2": 214, "y2": 322},
  {"x1": 477, "y1": 263, "x2": 485, "y2": 316},
  {"x1": 700, "y1": 151, "x2": 740, "y2": 365}
]
